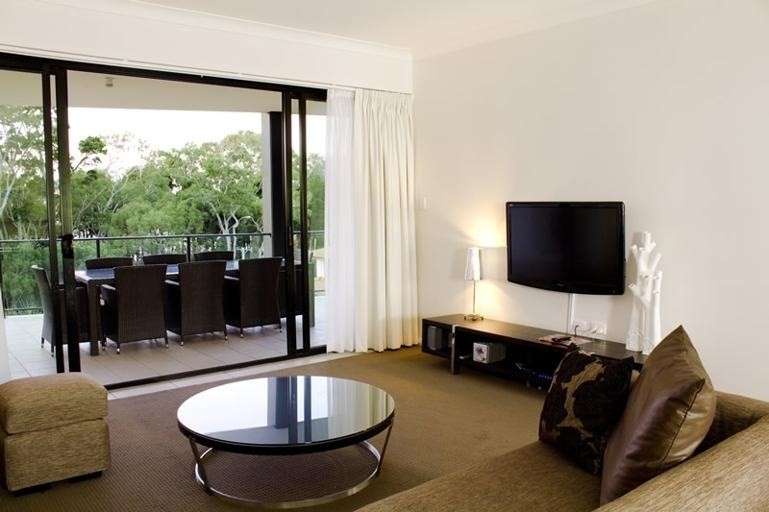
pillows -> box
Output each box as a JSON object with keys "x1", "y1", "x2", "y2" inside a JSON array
[
  {"x1": 538, "y1": 342, "x2": 634, "y2": 476},
  {"x1": 598, "y1": 324, "x2": 717, "y2": 506}
]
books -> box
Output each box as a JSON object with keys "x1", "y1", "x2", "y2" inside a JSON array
[{"x1": 536, "y1": 332, "x2": 593, "y2": 347}]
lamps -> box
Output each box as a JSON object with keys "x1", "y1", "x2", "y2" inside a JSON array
[{"x1": 465, "y1": 247, "x2": 485, "y2": 323}]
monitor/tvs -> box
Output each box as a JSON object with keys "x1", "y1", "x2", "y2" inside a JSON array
[{"x1": 505, "y1": 201, "x2": 626, "y2": 295}]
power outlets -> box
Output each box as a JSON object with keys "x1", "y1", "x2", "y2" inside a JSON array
[
  {"x1": 572, "y1": 318, "x2": 589, "y2": 333},
  {"x1": 590, "y1": 320, "x2": 607, "y2": 335}
]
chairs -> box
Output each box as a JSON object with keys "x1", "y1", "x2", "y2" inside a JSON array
[{"x1": 30, "y1": 251, "x2": 303, "y2": 357}]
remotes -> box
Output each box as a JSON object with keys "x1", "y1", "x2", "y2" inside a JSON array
[{"x1": 552, "y1": 336, "x2": 572, "y2": 342}]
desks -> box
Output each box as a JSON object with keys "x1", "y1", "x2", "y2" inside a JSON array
[{"x1": 422, "y1": 314, "x2": 648, "y2": 391}]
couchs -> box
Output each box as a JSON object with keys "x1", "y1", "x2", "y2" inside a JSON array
[
  {"x1": 351, "y1": 390, "x2": 768, "y2": 511},
  {"x1": 0, "y1": 371, "x2": 112, "y2": 498}
]
